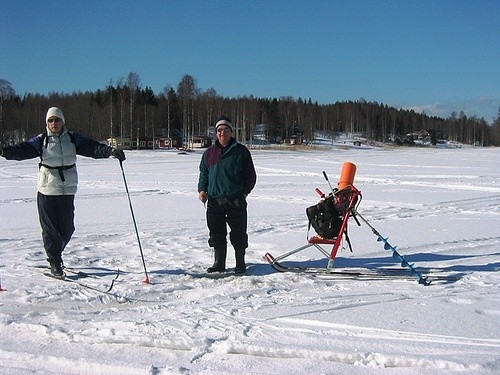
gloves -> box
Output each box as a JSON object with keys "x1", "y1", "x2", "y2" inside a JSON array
[{"x1": 112, "y1": 149, "x2": 126, "y2": 161}]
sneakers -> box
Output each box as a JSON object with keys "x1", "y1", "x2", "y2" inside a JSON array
[{"x1": 51, "y1": 261, "x2": 63, "y2": 275}]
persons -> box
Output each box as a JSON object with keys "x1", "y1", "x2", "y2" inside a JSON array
[
  {"x1": 0, "y1": 107, "x2": 126, "y2": 276},
  {"x1": 198, "y1": 120, "x2": 256, "y2": 274}
]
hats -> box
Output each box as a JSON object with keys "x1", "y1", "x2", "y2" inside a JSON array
[
  {"x1": 45, "y1": 106, "x2": 66, "y2": 124},
  {"x1": 216, "y1": 113, "x2": 233, "y2": 131}
]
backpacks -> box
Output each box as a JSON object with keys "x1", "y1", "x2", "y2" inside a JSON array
[{"x1": 306, "y1": 190, "x2": 362, "y2": 252}]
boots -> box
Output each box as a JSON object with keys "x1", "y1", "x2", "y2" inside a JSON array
[
  {"x1": 206, "y1": 242, "x2": 227, "y2": 272},
  {"x1": 235, "y1": 247, "x2": 245, "y2": 274}
]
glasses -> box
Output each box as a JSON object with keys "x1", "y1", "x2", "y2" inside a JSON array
[
  {"x1": 48, "y1": 118, "x2": 62, "y2": 122},
  {"x1": 216, "y1": 127, "x2": 230, "y2": 132}
]
